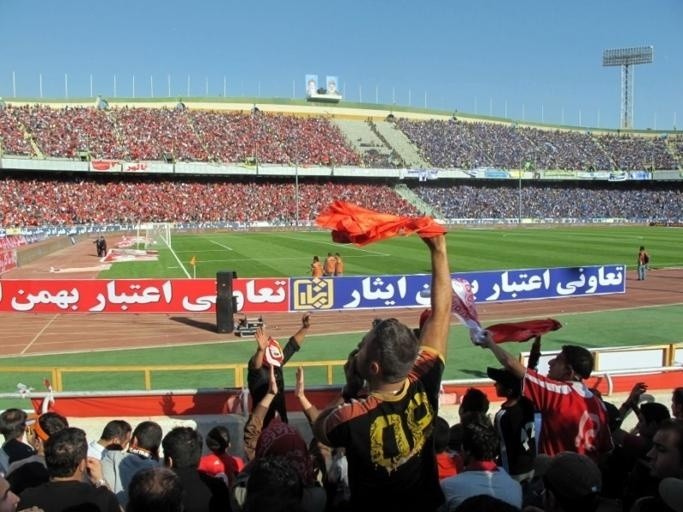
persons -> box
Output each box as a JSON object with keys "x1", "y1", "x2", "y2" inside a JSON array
[
  {"x1": 1, "y1": 174, "x2": 428, "y2": 229},
  {"x1": 2, "y1": 103, "x2": 408, "y2": 168},
  {"x1": 386, "y1": 114, "x2": 681, "y2": 171},
  {"x1": 307, "y1": 80, "x2": 317, "y2": 96},
  {"x1": 405, "y1": 178, "x2": 682, "y2": 218},
  {"x1": 1, "y1": 233, "x2": 681, "y2": 511},
  {"x1": 327, "y1": 80, "x2": 337, "y2": 95}
]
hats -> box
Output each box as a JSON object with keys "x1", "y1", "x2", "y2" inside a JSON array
[
  {"x1": 487, "y1": 366, "x2": 520, "y2": 386},
  {"x1": 255, "y1": 423, "x2": 308, "y2": 488},
  {"x1": 535, "y1": 453, "x2": 604, "y2": 502}
]
